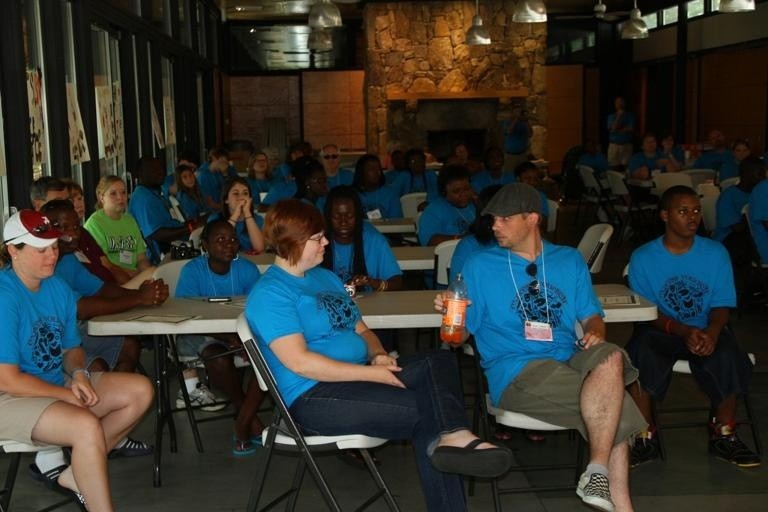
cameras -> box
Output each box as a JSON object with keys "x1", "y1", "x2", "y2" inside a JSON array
[
  {"x1": 342, "y1": 278, "x2": 357, "y2": 300},
  {"x1": 170, "y1": 239, "x2": 201, "y2": 261}
]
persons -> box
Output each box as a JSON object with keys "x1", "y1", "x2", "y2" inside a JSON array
[
  {"x1": 161, "y1": 151, "x2": 198, "y2": 195},
  {"x1": 174, "y1": 216, "x2": 268, "y2": 457},
  {"x1": 447, "y1": 140, "x2": 469, "y2": 163},
  {"x1": 659, "y1": 130, "x2": 687, "y2": 170},
  {"x1": 290, "y1": 158, "x2": 328, "y2": 206},
  {"x1": 24, "y1": 176, "x2": 131, "y2": 287},
  {"x1": 693, "y1": 129, "x2": 731, "y2": 169},
  {"x1": 279, "y1": 144, "x2": 304, "y2": 179},
  {"x1": 625, "y1": 185, "x2": 762, "y2": 470},
  {"x1": 514, "y1": 161, "x2": 548, "y2": 233},
  {"x1": 194, "y1": 147, "x2": 231, "y2": 211},
  {"x1": 241, "y1": 150, "x2": 272, "y2": 201},
  {"x1": 85, "y1": 175, "x2": 151, "y2": 280},
  {"x1": 417, "y1": 163, "x2": 480, "y2": 246},
  {"x1": 393, "y1": 147, "x2": 438, "y2": 196},
  {"x1": 1, "y1": 207, "x2": 146, "y2": 507},
  {"x1": 748, "y1": 180, "x2": 768, "y2": 261},
  {"x1": 433, "y1": 182, "x2": 651, "y2": 512},
  {"x1": 382, "y1": 148, "x2": 406, "y2": 184},
  {"x1": 606, "y1": 95, "x2": 634, "y2": 169},
  {"x1": 627, "y1": 132, "x2": 673, "y2": 179},
  {"x1": 321, "y1": 185, "x2": 403, "y2": 291},
  {"x1": 448, "y1": 183, "x2": 504, "y2": 283},
  {"x1": 574, "y1": 136, "x2": 611, "y2": 189},
  {"x1": 351, "y1": 154, "x2": 403, "y2": 224},
  {"x1": 318, "y1": 143, "x2": 352, "y2": 189},
  {"x1": 128, "y1": 155, "x2": 203, "y2": 260},
  {"x1": 713, "y1": 155, "x2": 766, "y2": 269},
  {"x1": 716, "y1": 138, "x2": 751, "y2": 181},
  {"x1": 257, "y1": 154, "x2": 307, "y2": 212},
  {"x1": 65, "y1": 179, "x2": 131, "y2": 284},
  {"x1": 207, "y1": 177, "x2": 265, "y2": 254},
  {"x1": 30, "y1": 199, "x2": 169, "y2": 494},
  {"x1": 474, "y1": 146, "x2": 516, "y2": 188},
  {"x1": 172, "y1": 163, "x2": 208, "y2": 220},
  {"x1": 248, "y1": 197, "x2": 514, "y2": 511}
]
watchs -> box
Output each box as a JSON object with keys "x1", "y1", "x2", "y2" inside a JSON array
[{"x1": 71, "y1": 367, "x2": 92, "y2": 378}]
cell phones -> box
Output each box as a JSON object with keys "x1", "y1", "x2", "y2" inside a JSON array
[{"x1": 208, "y1": 297, "x2": 232, "y2": 303}]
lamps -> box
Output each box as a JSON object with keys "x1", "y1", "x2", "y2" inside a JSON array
[
  {"x1": 466, "y1": 0, "x2": 491, "y2": 45},
  {"x1": 620, "y1": 0, "x2": 649, "y2": 39},
  {"x1": 719, "y1": 0, "x2": 756, "y2": 13},
  {"x1": 307, "y1": 32, "x2": 333, "y2": 50},
  {"x1": 594, "y1": 2, "x2": 607, "y2": 18},
  {"x1": 512, "y1": 1, "x2": 548, "y2": 22},
  {"x1": 308, "y1": 1, "x2": 343, "y2": 28}
]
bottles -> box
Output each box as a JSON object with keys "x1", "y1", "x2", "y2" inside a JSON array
[{"x1": 439, "y1": 271, "x2": 468, "y2": 345}]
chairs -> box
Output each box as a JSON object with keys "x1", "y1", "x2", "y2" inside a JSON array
[
  {"x1": 576, "y1": 223, "x2": 613, "y2": 274},
  {"x1": 153, "y1": 259, "x2": 276, "y2": 489},
  {"x1": 605, "y1": 170, "x2": 654, "y2": 247},
  {"x1": 622, "y1": 264, "x2": 764, "y2": 465},
  {"x1": 468, "y1": 334, "x2": 585, "y2": 512},
  {"x1": 168, "y1": 195, "x2": 185, "y2": 223},
  {"x1": 695, "y1": 183, "x2": 720, "y2": 239},
  {"x1": 434, "y1": 239, "x2": 460, "y2": 285},
  {"x1": 574, "y1": 165, "x2": 622, "y2": 233},
  {"x1": 236, "y1": 311, "x2": 400, "y2": 512},
  {"x1": 189, "y1": 226, "x2": 205, "y2": 249},
  {"x1": 652, "y1": 173, "x2": 692, "y2": 200},
  {"x1": 400, "y1": 192, "x2": 428, "y2": 221},
  {"x1": 0, "y1": 442, "x2": 77, "y2": 512}
]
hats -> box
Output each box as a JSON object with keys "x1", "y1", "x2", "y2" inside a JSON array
[
  {"x1": 3, "y1": 209, "x2": 72, "y2": 248},
  {"x1": 479, "y1": 180, "x2": 544, "y2": 218}
]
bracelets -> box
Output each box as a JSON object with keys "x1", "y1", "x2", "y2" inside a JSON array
[
  {"x1": 377, "y1": 280, "x2": 389, "y2": 291},
  {"x1": 229, "y1": 217, "x2": 237, "y2": 223},
  {"x1": 243, "y1": 215, "x2": 254, "y2": 220},
  {"x1": 664, "y1": 319, "x2": 672, "y2": 334},
  {"x1": 188, "y1": 223, "x2": 193, "y2": 233}
]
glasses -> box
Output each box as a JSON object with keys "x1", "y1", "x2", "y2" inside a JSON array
[
  {"x1": 308, "y1": 234, "x2": 324, "y2": 242},
  {"x1": 527, "y1": 263, "x2": 540, "y2": 298},
  {"x1": 3, "y1": 219, "x2": 60, "y2": 244},
  {"x1": 325, "y1": 154, "x2": 337, "y2": 159}
]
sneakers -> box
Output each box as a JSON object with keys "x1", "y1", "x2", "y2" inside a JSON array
[
  {"x1": 629, "y1": 426, "x2": 661, "y2": 467},
  {"x1": 709, "y1": 417, "x2": 761, "y2": 467},
  {"x1": 176, "y1": 382, "x2": 229, "y2": 412},
  {"x1": 575, "y1": 472, "x2": 615, "y2": 512}
]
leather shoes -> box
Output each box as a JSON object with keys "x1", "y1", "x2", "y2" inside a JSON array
[{"x1": 336, "y1": 446, "x2": 377, "y2": 469}]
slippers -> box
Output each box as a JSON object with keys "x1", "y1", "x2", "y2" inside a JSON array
[
  {"x1": 232, "y1": 433, "x2": 262, "y2": 445},
  {"x1": 52, "y1": 476, "x2": 88, "y2": 512},
  {"x1": 430, "y1": 438, "x2": 512, "y2": 478},
  {"x1": 107, "y1": 436, "x2": 153, "y2": 459},
  {"x1": 27, "y1": 463, "x2": 68, "y2": 490},
  {"x1": 231, "y1": 432, "x2": 255, "y2": 456}
]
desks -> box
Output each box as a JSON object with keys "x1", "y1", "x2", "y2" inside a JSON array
[
  {"x1": 121, "y1": 246, "x2": 433, "y2": 290},
  {"x1": 625, "y1": 178, "x2": 654, "y2": 187},
  {"x1": 371, "y1": 221, "x2": 416, "y2": 233},
  {"x1": 89, "y1": 284, "x2": 657, "y2": 331}
]
